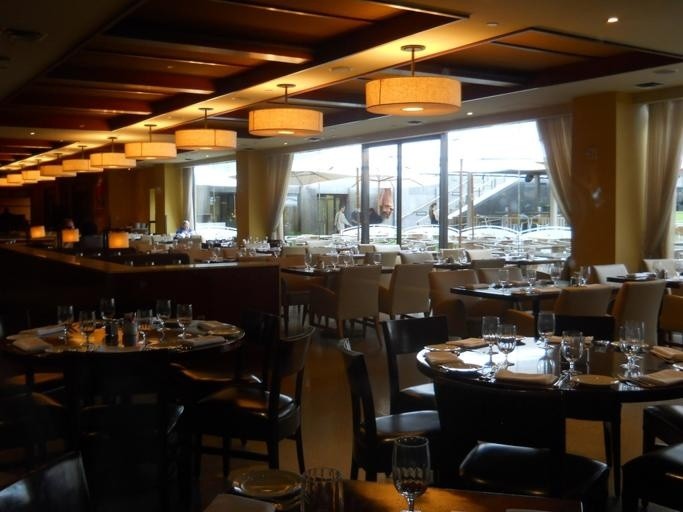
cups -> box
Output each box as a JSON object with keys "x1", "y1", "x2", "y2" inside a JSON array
[{"x1": 301, "y1": 465, "x2": 344, "y2": 512}]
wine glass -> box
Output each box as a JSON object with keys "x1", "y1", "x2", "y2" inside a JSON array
[
  {"x1": 122, "y1": 222, "x2": 383, "y2": 273},
  {"x1": 628, "y1": 258, "x2": 683, "y2": 281},
  {"x1": 392, "y1": 436, "x2": 433, "y2": 512},
  {"x1": 497, "y1": 267, "x2": 590, "y2": 294},
  {"x1": 405, "y1": 243, "x2": 573, "y2": 264},
  {"x1": 424, "y1": 311, "x2": 683, "y2": 390},
  {"x1": 4, "y1": 296, "x2": 243, "y2": 353}
]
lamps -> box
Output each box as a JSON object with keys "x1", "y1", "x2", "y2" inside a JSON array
[
  {"x1": 244, "y1": 84, "x2": 326, "y2": 139},
  {"x1": 90, "y1": 135, "x2": 136, "y2": 171},
  {"x1": 123, "y1": 123, "x2": 176, "y2": 162},
  {"x1": 361, "y1": 45, "x2": 463, "y2": 121},
  {"x1": 0, "y1": 143, "x2": 104, "y2": 189},
  {"x1": 173, "y1": 108, "x2": 238, "y2": 152}
]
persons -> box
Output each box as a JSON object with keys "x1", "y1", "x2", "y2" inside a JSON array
[
  {"x1": 428, "y1": 203, "x2": 438, "y2": 224},
  {"x1": 334, "y1": 204, "x2": 380, "y2": 233},
  {"x1": 176, "y1": 220, "x2": 192, "y2": 237}
]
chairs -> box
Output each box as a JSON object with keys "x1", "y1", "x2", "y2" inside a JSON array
[{"x1": 1, "y1": 222, "x2": 682, "y2": 512}]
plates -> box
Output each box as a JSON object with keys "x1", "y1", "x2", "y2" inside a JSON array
[{"x1": 232, "y1": 467, "x2": 306, "y2": 499}]
